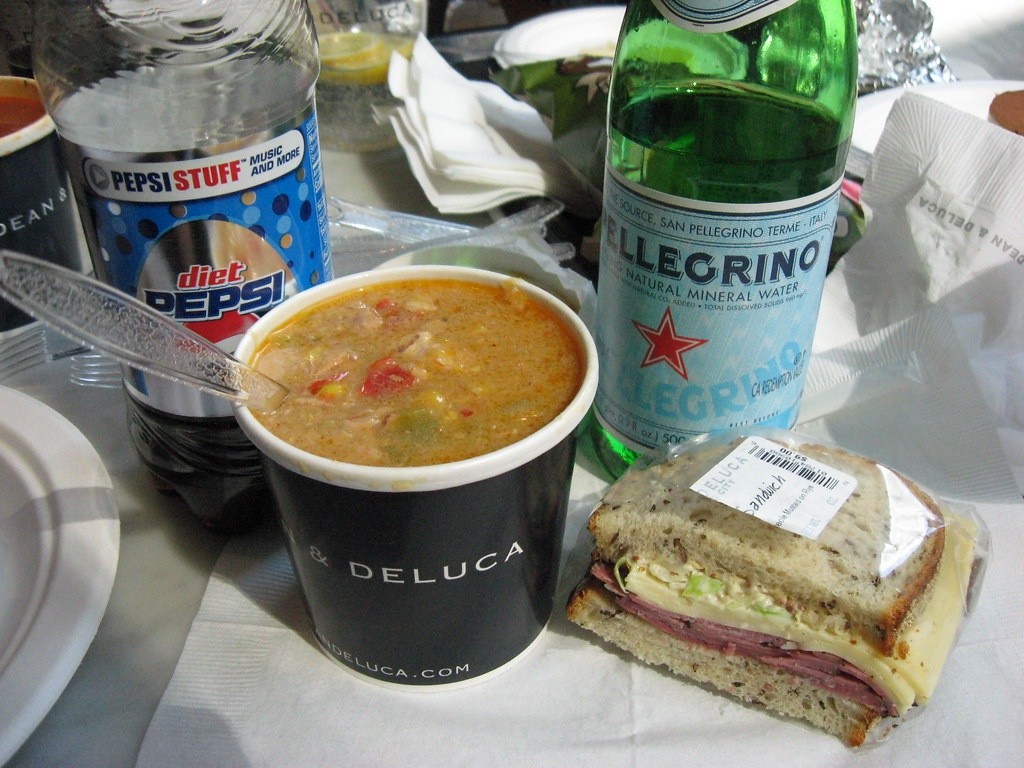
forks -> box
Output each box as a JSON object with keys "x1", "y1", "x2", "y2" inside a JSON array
[{"x1": 0, "y1": 323, "x2": 91, "y2": 381}]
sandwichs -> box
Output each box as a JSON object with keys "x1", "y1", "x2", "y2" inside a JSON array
[{"x1": 565, "y1": 436, "x2": 978, "y2": 751}]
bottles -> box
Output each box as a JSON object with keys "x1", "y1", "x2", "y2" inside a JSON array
[
  {"x1": 31, "y1": 1, "x2": 334, "y2": 538},
  {"x1": 592, "y1": 0, "x2": 859, "y2": 484}
]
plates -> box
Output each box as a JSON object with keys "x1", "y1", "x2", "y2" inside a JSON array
[{"x1": 0, "y1": 382, "x2": 120, "y2": 766}]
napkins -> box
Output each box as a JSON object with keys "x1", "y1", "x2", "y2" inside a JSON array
[{"x1": 368, "y1": 32, "x2": 557, "y2": 216}]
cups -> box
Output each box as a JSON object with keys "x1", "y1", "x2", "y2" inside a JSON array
[
  {"x1": 229, "y1": 263, "x2": 601, "y2": 694},
  {"x1": 0, "y1": 75, "x2": 84, "y2": 341}
]
